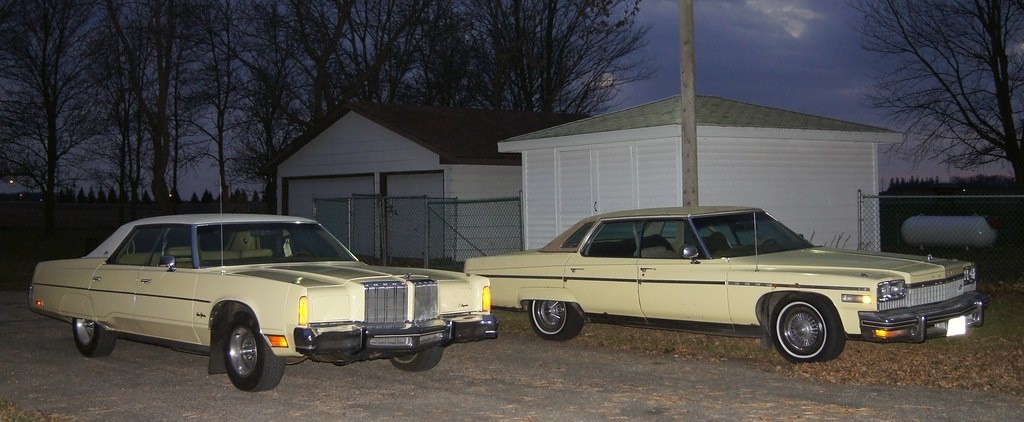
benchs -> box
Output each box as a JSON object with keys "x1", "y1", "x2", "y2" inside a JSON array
[
  {"x1": 581, "y1": 226, "x2": 675, "y2": 262},
  {"x1": 116, "y1": 244, "x2": 166, "y2": 265}
]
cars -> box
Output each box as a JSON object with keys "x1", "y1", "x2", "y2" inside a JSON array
[
  {"x1": 25, "y1": 208, "x2": 498, "y2": 400},
  {"x1": 461, "y1": 204, "x2": 986, "y2": 368}
]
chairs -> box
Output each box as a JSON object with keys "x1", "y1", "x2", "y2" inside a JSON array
[{"x1": 165, "y1": 244, "x2": 201, "y2": 264}]
